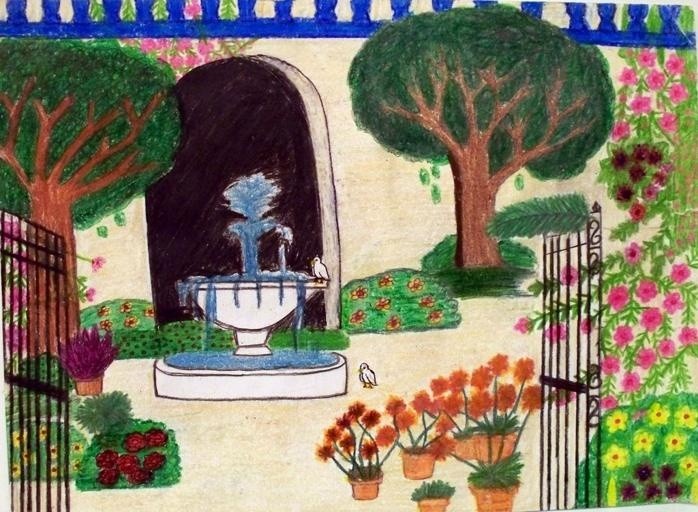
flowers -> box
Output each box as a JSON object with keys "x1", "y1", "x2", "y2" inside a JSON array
[
  {"x1": 315, "y1": 354, "x2": 543, "y2": 480},
  {"x1": 118, "y1": 0, "x2": 226, "y2": 80},
  {"x1": 1, "y1": 219, "x2": 107, "y2": 350}
]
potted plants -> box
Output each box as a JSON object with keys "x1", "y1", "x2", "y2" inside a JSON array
[
  {"x1": 410, "y1": 481, "x2": 455, "y2": 512},
  {"x1": 467, "y1": 450, "x2": 524, "y2": 512},
  {"x1": 56, "y1": 323, "x2": 119, "y2": 396}
]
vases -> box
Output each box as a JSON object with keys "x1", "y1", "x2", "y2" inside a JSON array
[{"x1": 348, "y1": 468, "x2": 384, "y2": 500}]
